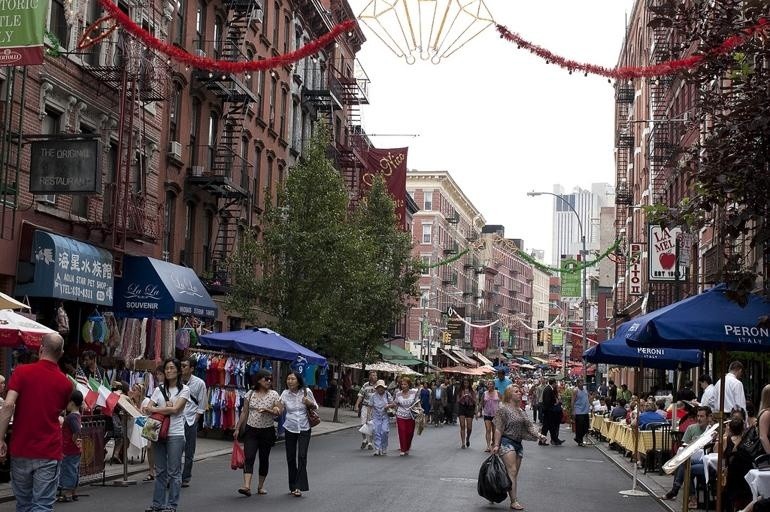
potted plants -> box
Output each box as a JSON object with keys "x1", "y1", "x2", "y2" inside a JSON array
[{"x1": 560, "y1": 386, "x2": 576, "y2": 432}]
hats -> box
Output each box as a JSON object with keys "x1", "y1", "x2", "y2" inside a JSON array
[{"x1": 374, "y1": 379, "x2": 388, "y2": 389}]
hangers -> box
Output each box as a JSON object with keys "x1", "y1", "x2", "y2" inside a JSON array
[
  {"x1": 206, "y1": 384, "x2": 247, "y2": 398},
  {"x1": 106, "y1": 364, "x2": 154, "y2": 377},
  {"x1": 188, "y1": 348, "x2": 259, "y2": 369}
]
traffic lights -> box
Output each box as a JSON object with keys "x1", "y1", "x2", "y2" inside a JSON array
[
  {"x1": 442, "y1": 331, "x2": 447, "y2": 344},
  {"x1": 546, "y1": 330, "x2": 552, "y2": 342},
  {"x1": 447, "y1": 331, "x2": 452, "y2": 344}
]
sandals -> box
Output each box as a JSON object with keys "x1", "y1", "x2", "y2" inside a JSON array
[
  {"x1": 258, "y1": 486, "x2": 267, "y2": 494},
  {"x1": 291, "y1": 489, "x2": 302, "y2": 497},
  {"x1": 142, "y1": 474, "x2": 155, "y2": 481},
  {"x1": 238, "y1": 486, "x2": 252, "y2": 497},
  {"x1": 510, "y1": 497, "x2": 525, "y2": 510}
]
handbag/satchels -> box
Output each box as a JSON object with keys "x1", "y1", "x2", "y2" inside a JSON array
[
  {"x1": 409, "y1": 399, "x2": 426, "y2": 436},
  {"x1": 231, "y1": 433, "x2": 246, "y2": 470},
  {"x1": 140, "y1": 385, "x2": 171, "y2": 443},
  {"x1": 736, "y1": 409, "x2": 768, "y2": 461},
  {"x1": 386, "y1": 391, "x2": 397, "y2": 418},
  {"x1": 358, "y1": 420, "x2": 376, "y2": 437},
  {"x1": 304, "y1": 387, "x2": 321, "y2": 428}
]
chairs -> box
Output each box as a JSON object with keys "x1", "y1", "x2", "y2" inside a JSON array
[
  {"x1": 696, "y1": 475, "x2": 704, "y2": 509},
  {"x1": 752, "y1": 454, "x2": 770, "y2": 468},
  {"x1": 704, "y1": 443, "x2": 734, "y2": 512}
]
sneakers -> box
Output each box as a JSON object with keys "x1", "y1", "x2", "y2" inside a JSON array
[
  {"x1": 55, "y1": 494, "x2": 79, "y2": 503},
  {"x1": 661, "y1": 492, "x2": 678, "y2": 501},
  {"x1": 399, "y1": 450, "x2": 409, "y2": 456},
  {"x1": 361, "y1": 439, "x2": 387, "y2": 456},
  {"x1": 461, "y1": 441, "x2": 496, "y2": 452},
  {"x1": 145, "y1": 504, "x2": 176, "y2": 512},
  {"x1": 538, "y1": 438, "x2": 585, "y2": 447}
]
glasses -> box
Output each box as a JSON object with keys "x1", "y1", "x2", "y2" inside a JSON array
[
  {"x1": 265, "y1": 376, "x2": 273, "y2": 382},
  {"x1": 181, "y1": 363, "x2": 189, "y2": 368}
]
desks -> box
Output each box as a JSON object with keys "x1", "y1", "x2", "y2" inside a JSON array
[
  {"x1": 744, "y1": 468, "x2": 770, "y2": 504},
  {"x1": 703, "y1": 453, "x2": 718, "y2": 484},
  {"x1": 589, "y1": 409, "x2": 685, "y2": 475}
]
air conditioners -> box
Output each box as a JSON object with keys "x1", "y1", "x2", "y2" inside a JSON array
[
  {"x1": 196, "y1": 50, "x2": 206, "y2": 58},
  {"x1": 252, "y1": 10, "x2": 264, "y2": 23},
  {"x1": 35, "y1": 195, "x2": 55, "y2": 205},
  {"x1": 169, "y1": 141, "x2": 182, "y2": 157},
  {"x1": 684, "y1": 112, "x2": 694, "y2": 131}
]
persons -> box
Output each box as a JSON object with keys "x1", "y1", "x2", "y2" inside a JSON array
[
  {"x1": 85, "y1": 351, "x2": 110, "y2": 381},
  {"x1": 355, "y1": 354, "x2": 770, "y2": 512},
  {"x1": 0, "y1": 332, "x2": 85, "y2": 512},
  {"x1": 272, "y1": 371, "x2": 320, "y2": 497},
  {"x1": 235, "y1": 369, "x2": 279, "y2": 496},
  {"x1": 103, "y1": 356, "x2": 208, "y2": 512}
]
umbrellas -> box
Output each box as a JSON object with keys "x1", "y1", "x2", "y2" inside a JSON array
[{"x1": 198, "y1": 326, "x2": 326, "y2": 367}]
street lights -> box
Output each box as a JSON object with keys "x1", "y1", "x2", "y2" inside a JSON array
[
  {"x1": 526, "y1": 189, "x2": 588, "y2": 384},
  {"x1": 497, "y1": 311, "x2": 527, "y2": 369},
  {"x1": 537, "y1": 300, "x2": 567, "y2": 370},
  {"x1": 420, "y1": 291, "x2": 465, "y2": 372}
]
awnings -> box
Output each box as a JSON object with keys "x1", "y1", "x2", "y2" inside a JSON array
[
  {"x1": 374, "y1": 345, "x2": 422, "y2": 366},
  {"x1": 440, "y1": 349, "x2": 493, "y2": 366}
]
